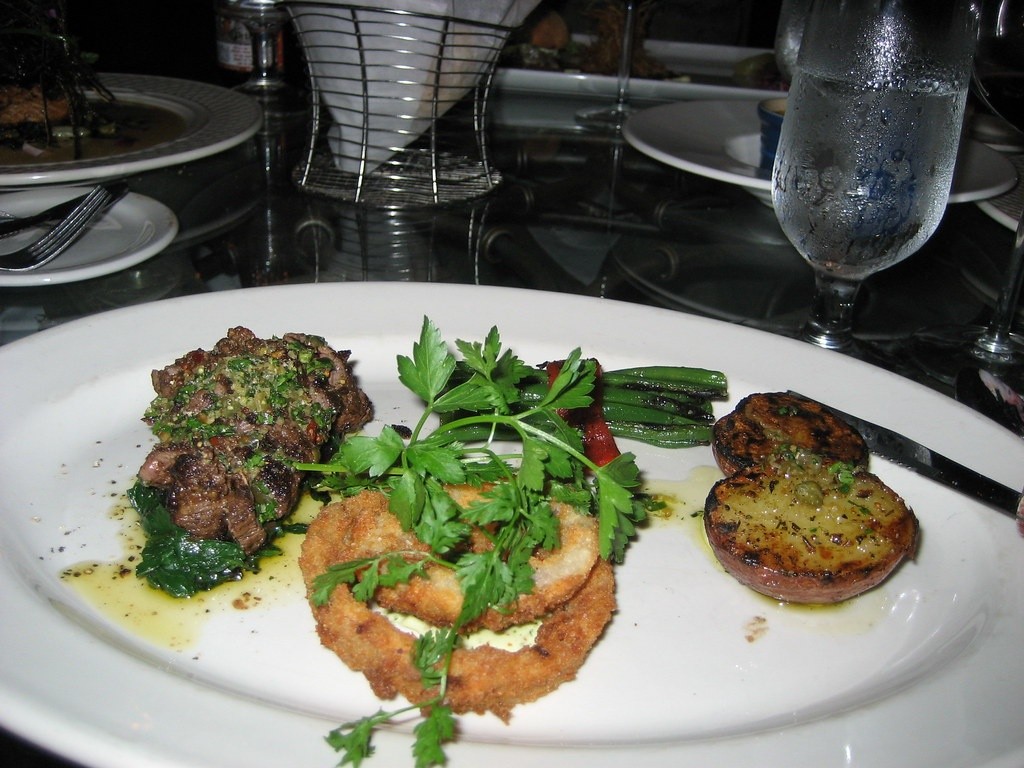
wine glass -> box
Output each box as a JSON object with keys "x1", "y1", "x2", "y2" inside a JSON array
[
  {"x1": 226, "y1": 0, "x2": 293, "y2": 104},
  {"x1": 770, "y1": 1, "x2": 987, "y2": 368},
  {"x1": 573, "y1": 1, "x2": 663, "y2": 135}
]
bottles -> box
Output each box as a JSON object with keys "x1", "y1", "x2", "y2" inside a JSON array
[{"x1": 213, "y1": 0, "x2": 278, "y2": 70}]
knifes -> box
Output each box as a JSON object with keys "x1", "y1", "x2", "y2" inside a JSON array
[
  {"x1": 780, "y1": 389, "x2": 1023, "y2": 526},
  {"x1": 1, "y1": 181, "x2": 133, "y2": 239}
]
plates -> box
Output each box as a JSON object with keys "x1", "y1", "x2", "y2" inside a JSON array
[
  {"x1": 616, "y1": 100, "x2": 1018, "y2": 208},
  {"x1": 0, "y1": 67, "x2": 264, "y2": 186},
  {"x1": 480, "y1": 25, "x2": 791, "y2": 103},
  {"x1": 909, "y1": 213, "x2": 1024, "y2": 386},
  {"x1": 0, "y1": 280, "x2": 1024, "y2": 768},
  {"x1": 972, "y1": 165, "x2": 1024, "y2": 237},
  {"x1": 0, "y1": 185, "x2": 178, "y2": 288},
  {"x1": 959, "y1": 110, "x2": 1024, "y2": 152}
]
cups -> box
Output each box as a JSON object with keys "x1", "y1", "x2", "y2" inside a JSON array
[{"x1": 774, "y1": 0, "x2": 820, "y2": 91}]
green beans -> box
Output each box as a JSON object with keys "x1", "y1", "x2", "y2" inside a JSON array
[{"x1": 432, "y1": 365, "x2": 727, "y2": 448}]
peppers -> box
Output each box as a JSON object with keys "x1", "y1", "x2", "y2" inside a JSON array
[{"x1": 546, "y1": 357, "x2": 622, "y2": 464}]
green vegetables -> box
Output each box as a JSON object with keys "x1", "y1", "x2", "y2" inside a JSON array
[
  {"x1": 127, "y1": 340, "x2": 342, "y2": 598},
  {"x1": 295, "y1": 313, "x2": 640, "y2": 768}
]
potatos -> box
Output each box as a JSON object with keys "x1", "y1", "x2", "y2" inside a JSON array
[{"x1": 703, "y1": 390, "x2": 920, "y2": 603}]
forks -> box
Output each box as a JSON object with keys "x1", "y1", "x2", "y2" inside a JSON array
[{"x1": 0, "y1": 186, "x2": 112, "y2": 273}]
